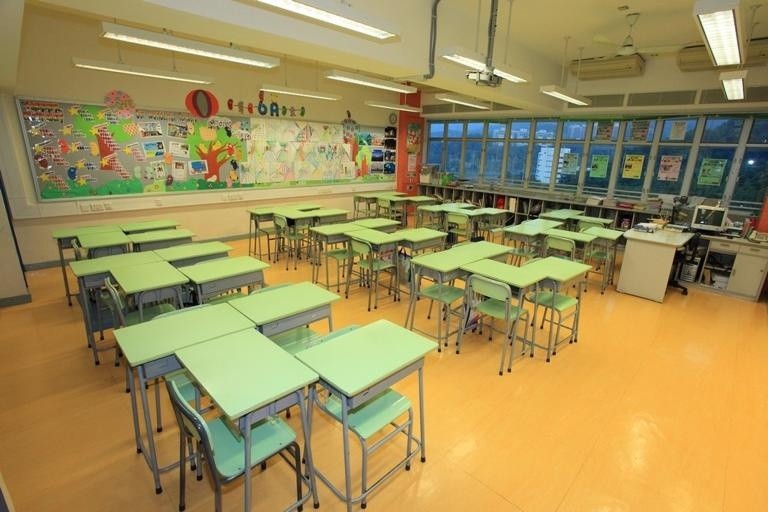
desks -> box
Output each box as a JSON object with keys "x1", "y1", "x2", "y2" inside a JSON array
[
  {"x1": 354, "y1": 192, "x2": 438, "y2": 228},
  {"x1": 111, "y1": 282, "x2": 441, "y2": 511},
  {"x1": 615, "y1": 221, "x2": 698, "y2": 304},
  {"x1": 50, "y1": 219, "x2": 270, "y2": 367},
  {"x1": 694, "y1": 227, "x2": 767, "y2": 303},
  {"x1": 248, "y1": 204, "x2": 448, "y2": 312}
]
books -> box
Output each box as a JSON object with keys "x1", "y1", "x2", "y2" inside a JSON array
[{"x1": 585, "y1": 196, "x2": 674, "y2": 215}]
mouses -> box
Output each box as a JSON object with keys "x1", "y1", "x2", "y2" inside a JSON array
[{"x1": 727, "y1": 236, "x2": 733, "y2": 239}]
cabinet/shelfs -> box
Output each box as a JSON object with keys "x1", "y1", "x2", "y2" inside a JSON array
[{"x1": 414, "y1": 181, "x2": 674, "y2": 253}]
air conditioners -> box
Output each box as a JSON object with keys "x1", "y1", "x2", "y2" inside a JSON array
[
  {"x1": 568, "y1": 54, "x2": 647, "y2": 83},
  {"x1": 673, "y1": 38, "x2": 768, "y2": 75}
]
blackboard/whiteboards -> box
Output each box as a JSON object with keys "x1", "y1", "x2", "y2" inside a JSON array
[{"x1": 15, "y1": 95, "x2": 397, "y2": 203}]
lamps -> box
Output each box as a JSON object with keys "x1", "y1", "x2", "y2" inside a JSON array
[
  {"x1": 98, "y1": 20, "x2": 279, "y2": 69},
  {"x1": 75, "y1": 63, "x2": 213, "y2": 86},
  {"x1": 436, "y1": 94, "x2": 487, "y2": 111},
  {"x1": 691, "y1": 0, "x2": 755, "y2": 70},
  {"x1": 262, "y1": 1, "x2": 397, "y2": 41},
  {"x1": 444, "y1": 54, "x2": 527, "y2": 84},
  {"x1": 259, "y1": 84, "x2": 342, "y2": 101},
  {"x1": 364, "y1": 100, "x2": 421, "y2": 112},
  {"x1": 715, "y1": 64, "x2": 749, "y2": 103},
  {"x1": 326, "y1": 69, "x2": 418, "y2": 95},
  {"x1": 540, "y1": 35, "x2": 596, "y2": 107}
]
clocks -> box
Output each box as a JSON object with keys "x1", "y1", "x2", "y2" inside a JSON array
[{"x1": 388, "y1": 112, "x2": 399, "y2": 126}]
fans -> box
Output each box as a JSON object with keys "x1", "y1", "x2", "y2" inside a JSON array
[{"x1": 591, "y1": 12, "x2": 685, "y2": 59}]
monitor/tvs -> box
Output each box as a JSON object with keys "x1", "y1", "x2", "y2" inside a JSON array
[{"x1": 690, "y1": 205, "x2": 728, "y2": 236}]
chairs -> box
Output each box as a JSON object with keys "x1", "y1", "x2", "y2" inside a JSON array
[
  {"x1": 403, "y1": 235, "x2": 594, "y2": 375},
  {"x1": 667, "y1": 230, "x2": 702, "y2": 297}
]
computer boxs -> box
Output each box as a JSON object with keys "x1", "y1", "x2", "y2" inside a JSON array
[{"x1": 679, "y1": 255, "x2": 701, "y2": 283}]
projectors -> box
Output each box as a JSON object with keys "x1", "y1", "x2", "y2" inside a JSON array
[{"x1": 467, "y1": 72, "x2": 502, "y2": 85}]
